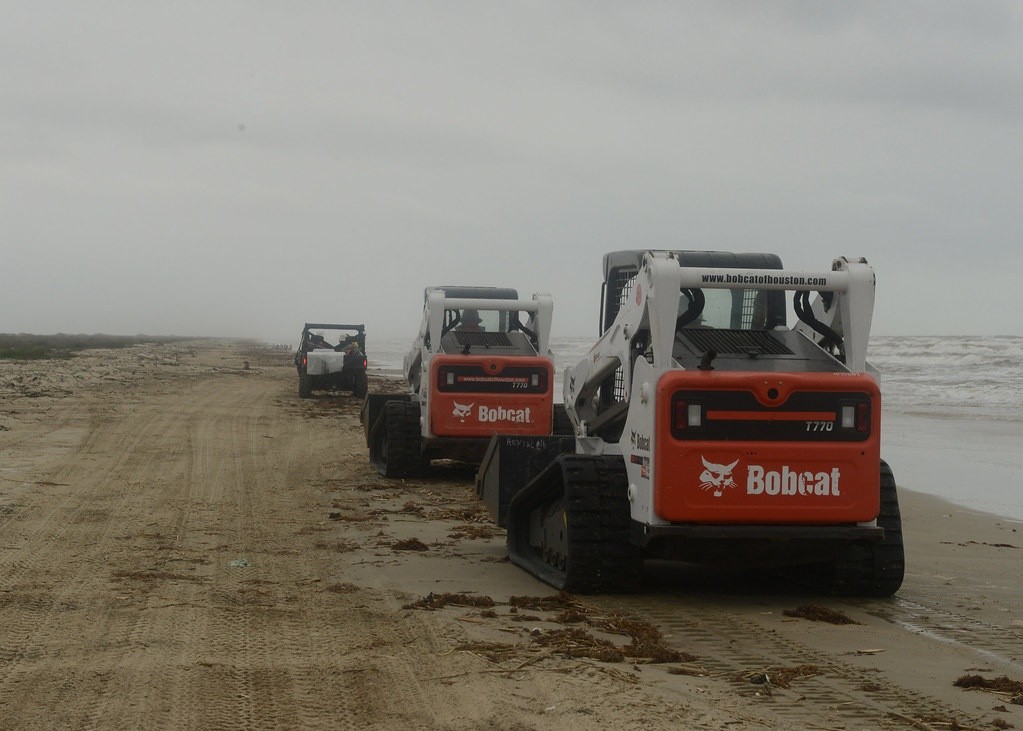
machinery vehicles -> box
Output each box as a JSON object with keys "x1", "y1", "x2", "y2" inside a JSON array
[
  {"x1": 361, "y1": 286, "x2": 574, "y2": 477},
  {"x1": 503, "y1": 249, "x2": 904, "y2": 598}
]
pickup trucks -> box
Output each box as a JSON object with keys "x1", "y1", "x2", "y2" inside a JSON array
[{"x1": 294, "y1": 322, "x2": 369, "y2": 398}]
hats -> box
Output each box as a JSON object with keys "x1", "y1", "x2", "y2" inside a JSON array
[
  {"x1": 339, "y1": 333, "x2": 348, "y2": 341},
  {"x1": 459, "y1": 310, "x2": 483, "y2": 322},
  {"x1": 314, "y1": 330, "x2": 324, "y2": 335}
]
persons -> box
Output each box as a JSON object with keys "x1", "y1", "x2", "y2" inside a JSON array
[
  {"x1": 678, "y1": 287, "x2": 713, "y2": 329},
  {"x1": 455, "y1": 310, "x2": 485, "y2": 332},
  {"x1": 311, "y1": 333, "x2": 346, "y2": 352}
]
